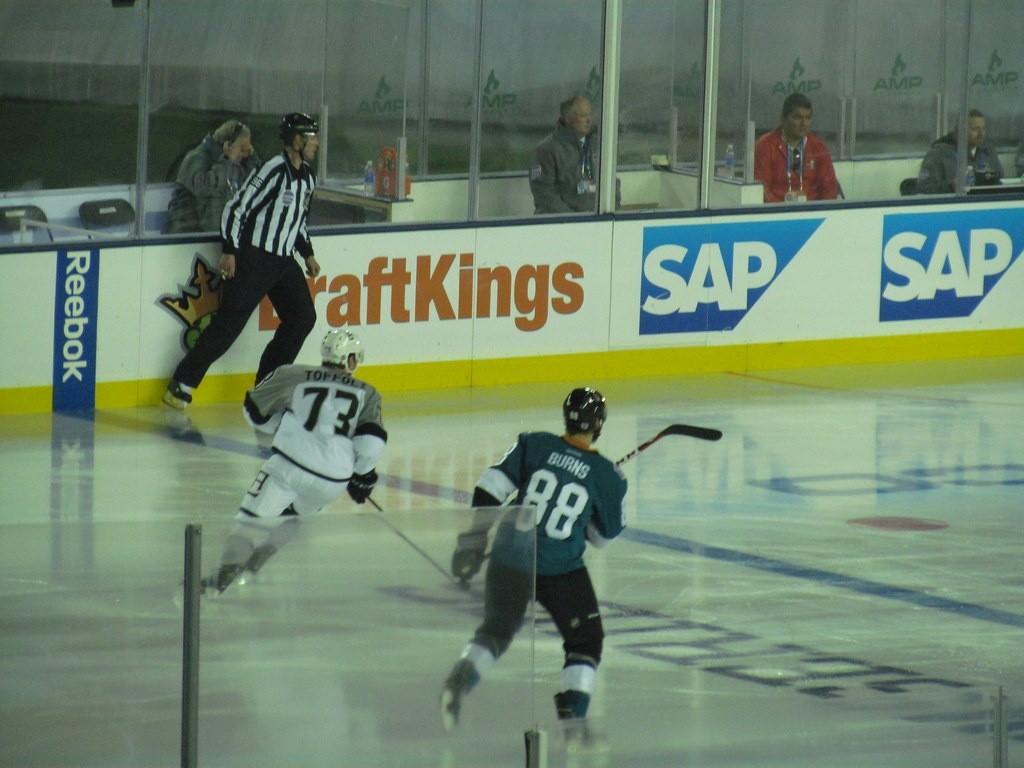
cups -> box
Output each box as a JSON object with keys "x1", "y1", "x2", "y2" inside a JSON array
[{"x1": 1000, "y1": 176, "x2": 1021, "y2": 185}]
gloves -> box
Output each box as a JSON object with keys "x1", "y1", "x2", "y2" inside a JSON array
[{"x1": 452, "y1": 530, "x2": 488, "y2": 581}]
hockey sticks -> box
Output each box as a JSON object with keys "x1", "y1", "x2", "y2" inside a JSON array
[
  {"x1": 483, "y1": 424, "x2": 724, "y2": 561},
  {"x1": 358, "y1": 492, "x2": 468, "y2": 591}
]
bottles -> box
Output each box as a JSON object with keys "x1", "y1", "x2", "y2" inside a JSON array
[
  {"x1": 966, "y1": 166, "x2": 975, "y2": 187},
  {"x1": 364, "y1": 161, "x2": 375, "y2": 198},
  {"x1": 724, "y1": 145, "x2": 734, "y2": 179},
  {"x1": 382, "y1": 152, "x2": 410, "y2": 178}
]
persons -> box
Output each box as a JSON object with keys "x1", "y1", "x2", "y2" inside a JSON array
[
  {"x1": 528, "y1": 97, "x2": 622, "y2": 214},
  {"x1": 753, "y1": 93, "x2": 837, "y2": 202},
  {"x1": 914, "y1": 109, "x2": 1003, "y2": 195},
  {"x1": 162, "y1": 112, "x2": 321, "y2": 409},
  {"x1": 162, "y1": 119, "x2": 260, "y2": 234},
  {"x1": 439, "y1": 387, "x2": 628, "y2": 755},
  {"x1": 176, "y1": 330, "x2": 388, "y2": 600}
]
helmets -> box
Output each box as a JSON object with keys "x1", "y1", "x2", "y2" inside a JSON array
[
  {"x1": 320, "y1": 330, "x2": 364, "y2": 373},
  {"x1": 279, "y1": 113, "x2": 319, "y2": 139},
  {"x1": 563, "y1": 387, "x2": 607, "y2": 443}
]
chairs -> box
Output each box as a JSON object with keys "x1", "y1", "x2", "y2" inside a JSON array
[
  {"x1": 80, "y1": 198, "x2": 139, "y2": 242},
  {"x1": 0, "y1": 205, "x2": 54, "y2": 249}
]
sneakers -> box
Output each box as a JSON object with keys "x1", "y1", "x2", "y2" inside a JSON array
[
  {"x1": 438, "y1": 658, "x2": 480, "y2": 734},
  {"x1": 239, "y1": 545, "x2": 277, "y2": 584},
  {"x1": 163, "y1": 377, "x2": 193, "y2": 409},
  {"x1": 554, "y1": 692, "x2": 611, "y2": 754},
  {"x1": 200, "y1": 563, "x2": 240, "y2": 599}
]
